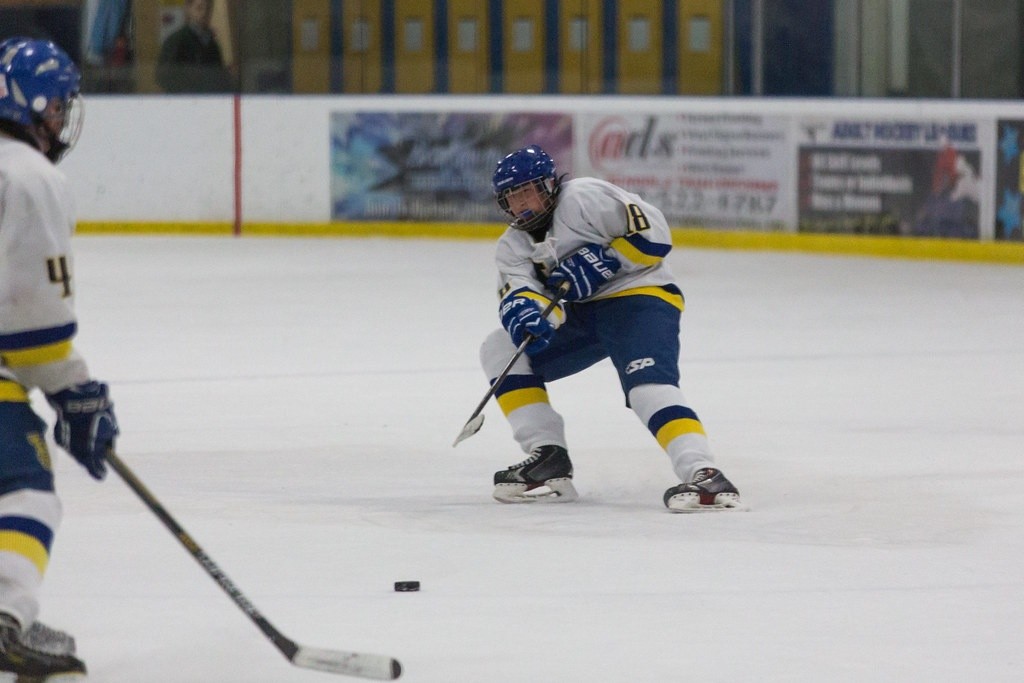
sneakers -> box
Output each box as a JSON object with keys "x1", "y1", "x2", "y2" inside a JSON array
[
  {"x1": 0, "y1": 610, "x2": 89, "y2": 683},
  {"x1": 663, "y1": 467, "x2": 741, "y2": 513},
  {"x1": 492, "y1": 444, "x2": 578, "y2": 503}
]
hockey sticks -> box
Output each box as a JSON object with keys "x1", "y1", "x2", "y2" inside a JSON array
[
  {"x1": 451, "y1": 280, "x2": 573, "y2": 448},
  {"x1": 106, "y1": 444, "x2": 405, "y2": 682}
]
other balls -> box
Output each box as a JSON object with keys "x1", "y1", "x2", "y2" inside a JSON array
[{"x1": 394, "y1": 580, "x2": 421, "y2": 593}]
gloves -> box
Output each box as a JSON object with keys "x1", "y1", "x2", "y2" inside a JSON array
[
  {"x1": 44, "y1": 380, "x2": 121, "y2": 481},
  {"x1": 545, "y1": 243, "x2": 622, "y2": 301},
  {"x1": 497, "y1": 296, "x2": 557, "y2": 354}
]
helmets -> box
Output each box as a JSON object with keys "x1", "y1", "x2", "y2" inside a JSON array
[
  {"x1": 490, "y1": 144, "x2": 559, "y2": 232},
  {"x1": 0, "y1": 36, "x2": 86, "y2": 164}
]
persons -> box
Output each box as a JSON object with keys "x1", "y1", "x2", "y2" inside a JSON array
[
  {"x1": 155, "y1": 0, "x2": 229, "y2": 94},
  {"x1": 478, "y1": 147, "x2": 752, "y2": 514},
  {"x1": 0, "y1": 38, "x2": 121, "y2": 683}
]
